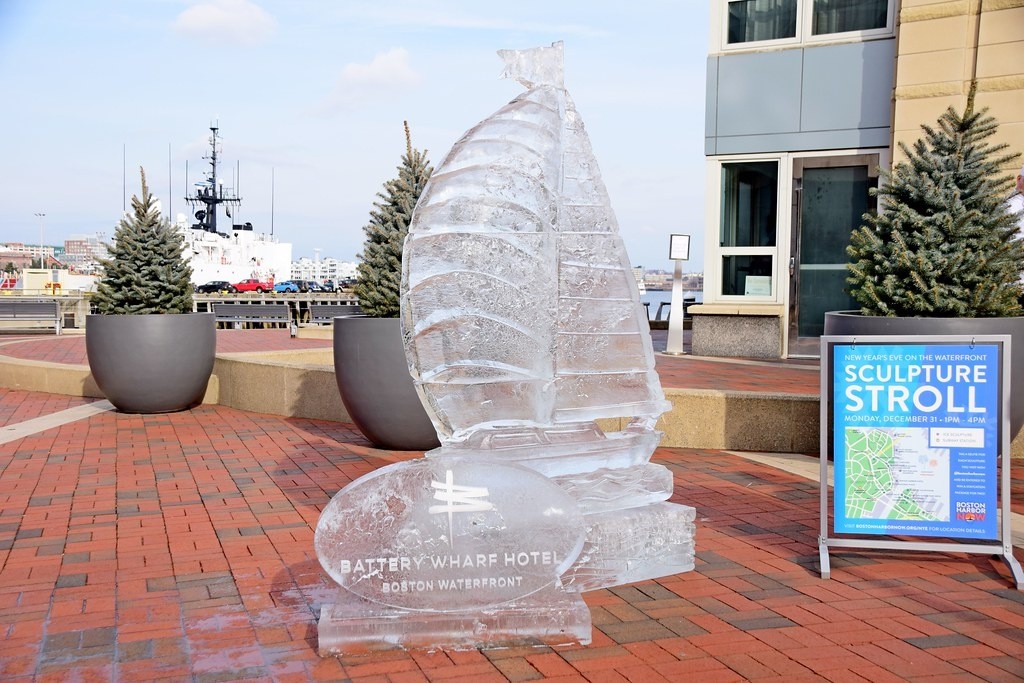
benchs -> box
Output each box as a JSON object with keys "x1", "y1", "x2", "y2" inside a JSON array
[
  {"x1": 212, "y1": 303, "x2": 296, "y2": 340},
  {"x1": 309, "y1": 306, "x2": 373, "y2": 325},
  {"x1": 0, "y1": 301, "x2": 63, "y2": 336}
]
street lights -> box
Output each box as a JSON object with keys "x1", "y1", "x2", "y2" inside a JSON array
[{"x1": 34, "y1": 213, "x2": 46, "y2": 270}]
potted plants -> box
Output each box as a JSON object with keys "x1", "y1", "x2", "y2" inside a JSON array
[
  {"x1": 822, "y1": 76, "x2": 1024, "y2": 459},
  {"x1": 332, "y1": 119, "x2": 442, "y2": 452},
  {"x1": 84, "y1": 167, "x2": 218, "y2": 416}
]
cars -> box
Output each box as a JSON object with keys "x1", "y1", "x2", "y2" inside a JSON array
[
  {"x1": 273, "y1": 282, "x2": 300, "y2": 293},
  {"x1": 197, "y1": 281, "x2": 235, "y2": 293},
  {"x1": 285, "y1": 278, "x2": 357, "y2": 293},
  {"x1": 231, "y1": 279, "x2": 274, "y2": 294}
]
